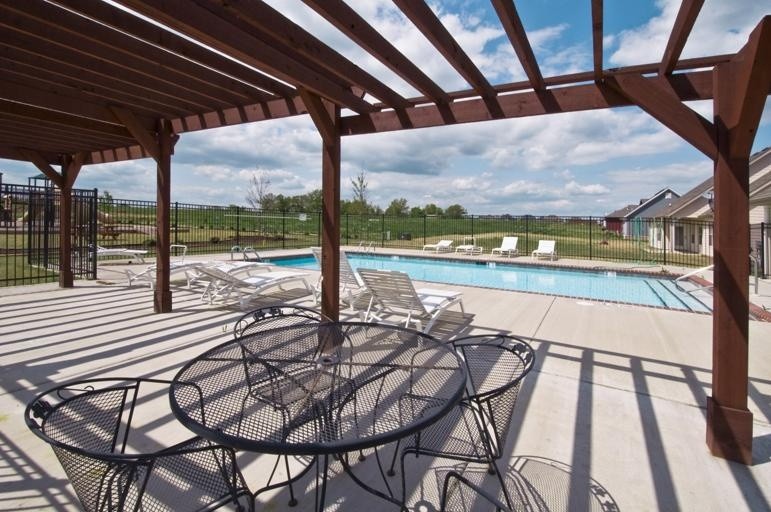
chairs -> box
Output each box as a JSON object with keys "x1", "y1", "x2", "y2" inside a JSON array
[
  {"x1": 233, "y1": 304, "x2": 368, "y2": 508},
  {"x1": 440, "y1": 470, "x2": 510, "y2": 512},
  {"x1": 422, "y1": 237, "x2": 558, "y2": 264},
  {"x1": 356, "y1": 268, "x2": 470, "y2": 345},
  {"x1": 310, "y1": 245, "x2": 369, "y2": 311},
  {"x1": 85, "y1": 240, "x2": 312, "y2": 309},
  {"x1": 388, "y1": 335, "x2": 538, "y2": 510},
  {"x1": 24, "y1": 378, "x2": 253, "y2": 512}
]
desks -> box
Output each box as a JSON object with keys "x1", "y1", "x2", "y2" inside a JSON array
[{"x1": 170, "y1": 319, "x2": 469, "y2": 512}]
